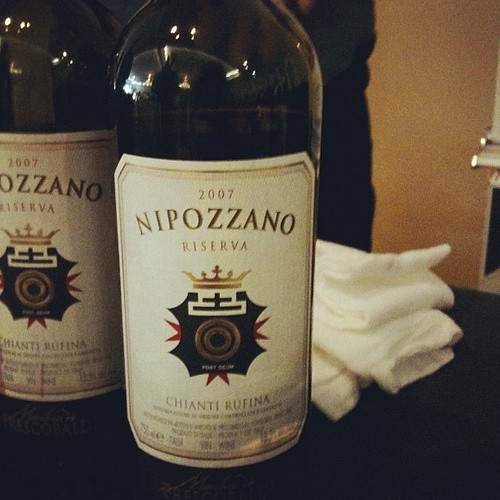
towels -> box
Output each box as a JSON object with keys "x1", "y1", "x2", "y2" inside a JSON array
[{"x1": 310, "y1": 238, "x2": 467, "y2": 426}]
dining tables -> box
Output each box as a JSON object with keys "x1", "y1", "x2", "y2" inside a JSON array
[{"x1": 1, "y1": 237, "x2": 500, "y2": 500}]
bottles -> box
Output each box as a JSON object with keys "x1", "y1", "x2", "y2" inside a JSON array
[
  {"x1": 107, "y1": 1, "x2": 324, "y2": 500},
  {"x1": 1, "y1": 0, "x2": 124, "y2": 500}
]
